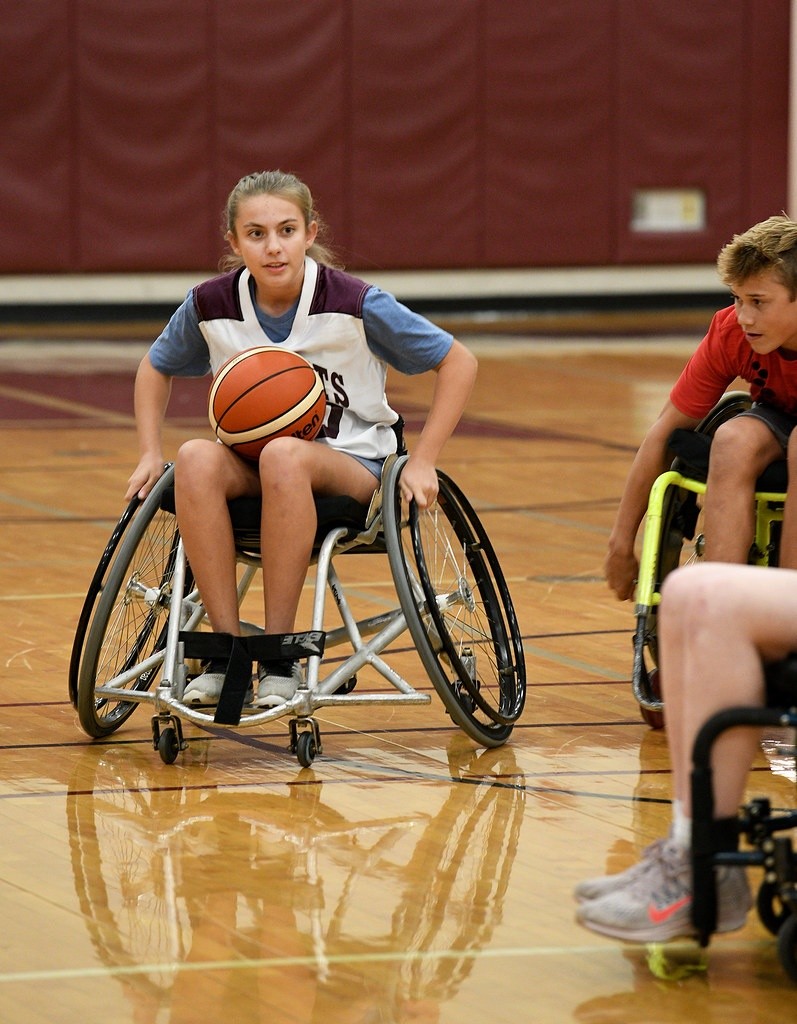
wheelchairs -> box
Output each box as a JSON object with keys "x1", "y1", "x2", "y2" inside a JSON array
[
  {"x1": 64, "y1": 447, "x2": 528, "y2": 769},
  {"x1": 618, "y1": 385, "x2": 796, "y2": 992}
]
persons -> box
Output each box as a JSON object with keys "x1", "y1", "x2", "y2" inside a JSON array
[
  {"x1": 127, "y1": 171, "x2": 478, "y2": 708},
  {"x1": 577, "y1": 561, "x2": 797, "y2": 942},
  {"x1": 603, "y1": 216, "x2": 797, "y2": 599}
]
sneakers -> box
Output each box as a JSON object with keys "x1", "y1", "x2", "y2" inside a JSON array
[
  {"x1": 257, "y1": 659, "x2": 303, "y2": 706},
  {"x1": 572, "y1": 843, "x2": 753, "y2": 912},
  {"x1": 183, "y1": 658, "x2": 254, "y2": 705},
  {"x1": 576, "y1": 842, "x2": 745, "y2": 942}
]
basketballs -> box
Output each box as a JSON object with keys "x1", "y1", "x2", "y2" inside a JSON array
[{"x1": 205, "y1": 343, "x2": 327, "y2": 460}]
